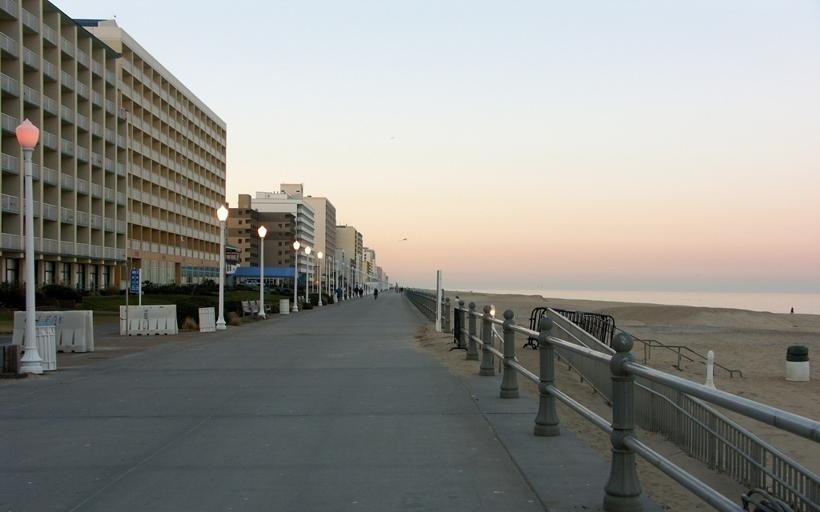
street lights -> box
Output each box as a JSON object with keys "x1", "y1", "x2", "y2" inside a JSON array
[
  {"x1": 215, "y1": 204, "x2": 229, "y2": 330},
  {"x1": 317, "y1": 250, "x2": 323, "y2": 307},
  {"x1": 327, "y1": 256, "x2": 393, "y2": 303},
  {"x1": 292, "y1": 240, "x2": 300, "y2": 312},
  {"x1": 257, "y1": 225, "x2": 267, "y2": 320},
  {"x1": 13, "y1": 118, "x2": 45, "y2": 377},
  {"x1": 303, "y1": 246, "x2": 312, "y2": 310}
]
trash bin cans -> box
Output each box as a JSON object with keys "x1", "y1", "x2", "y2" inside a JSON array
[
  {"x1": 198, "y1": 307, "x2": 216, "y2": 333},
  {"x1": 37, "y1": 327, "x2": 56, "y2": 370},
  {"x1": 279, "y1": 299, "x2": 289, "y2": 315},
  {"x1": 784, "y1": 345, "x2": 811, "y2": 382}
]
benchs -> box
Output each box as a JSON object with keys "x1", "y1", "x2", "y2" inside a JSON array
[{"x1": 242, "y1": 295, "x2": 305, "y2": 319}]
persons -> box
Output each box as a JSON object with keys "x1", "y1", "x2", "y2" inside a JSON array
[
  {"x1": 335, "y1": 284, "x2": 363, "y2": 303},
  {"x1": 372, "y1": 287, "x2": 378, "y2": 302},
  {"x1": 454, "y1": 295, "x2": 461, "y2": 306},
  {"x1": 379, "y1": 287, "x2": 405, "y2": 296},
  {"x1": 790, "y1": 306, "x2": 794, "y2": 315}
]
